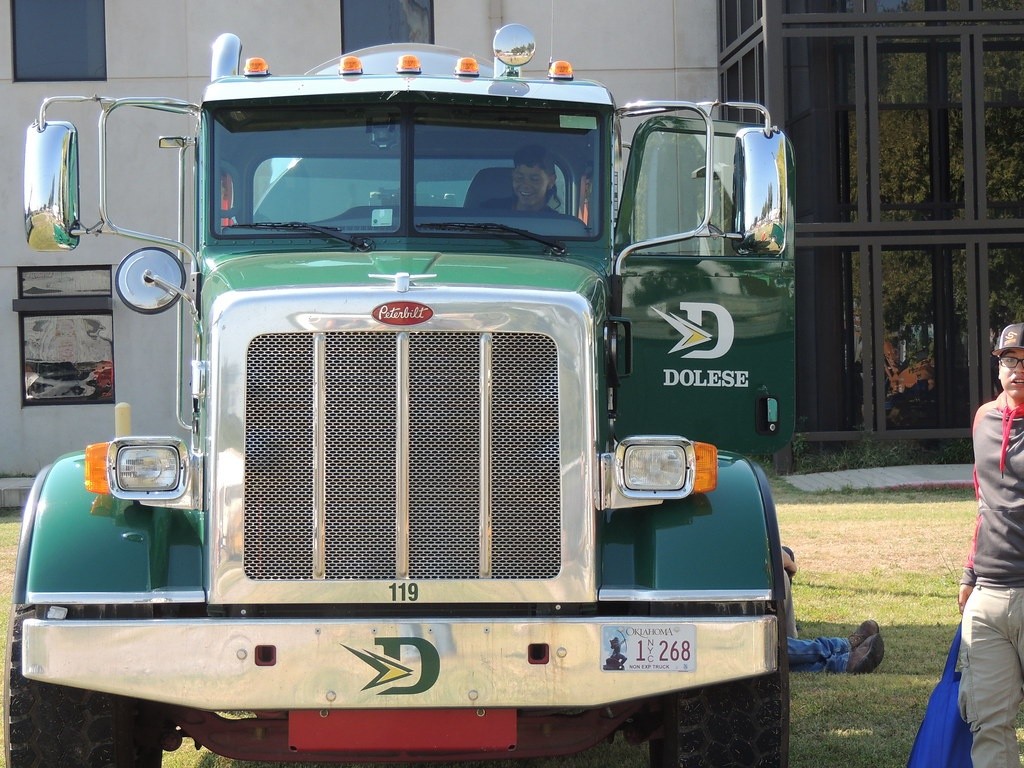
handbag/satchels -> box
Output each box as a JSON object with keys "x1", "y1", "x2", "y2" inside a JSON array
[{"x1": 906, "y1": 620, "x2": 974, "y2": 767}]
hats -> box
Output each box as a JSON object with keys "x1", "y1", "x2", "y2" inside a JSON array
[{"x1": 991, "y1": 322, "x2": 1024, "y2": 357}]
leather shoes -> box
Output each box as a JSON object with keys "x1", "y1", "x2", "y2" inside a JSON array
[
  {"x1": 846, "y1": 634, "x2": 885, "y2": 674},
  {"x1": 849, "y1": 620, "x2": 879, "y2": 648}
]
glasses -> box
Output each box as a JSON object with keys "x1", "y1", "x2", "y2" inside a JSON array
[{"x1": 999, "y1": 357, "x2": 1024, "y2": 368}]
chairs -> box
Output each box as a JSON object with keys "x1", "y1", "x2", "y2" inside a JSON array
[{"x1": 462, "y1": 168, "x2": 517, "y2": 211}]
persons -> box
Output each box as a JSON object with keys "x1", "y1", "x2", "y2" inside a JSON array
[
  {"x1": 777, "y1": 546, "x2": 800, "y2": 644},
  {"x1": 778, "y1": 619, "x2": 886, "y2": 678},
  {"x1": 957, "y1": 321, "x2": 1024, "y2": 768},
  {"x1": 466, "y1": 143, "x2": 563, "y2": 218}
]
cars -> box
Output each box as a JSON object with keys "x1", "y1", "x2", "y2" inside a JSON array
[{"x1": 9, "y1": 23, "x2": 807, "y2": 763}]
